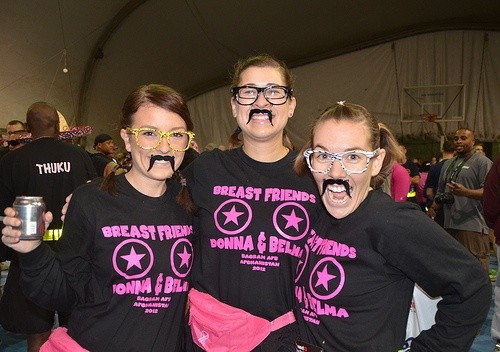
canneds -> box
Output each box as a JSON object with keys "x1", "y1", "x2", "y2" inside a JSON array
[{"x1": 12, "y1": 195, "x2": 46, "y2": 239}]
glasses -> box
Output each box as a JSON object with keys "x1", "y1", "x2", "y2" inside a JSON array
[
  {"x1": 23, "y1": 138, "x2": 33, "y2": 143},
  {"x1": 302, "y1": 147, "x2": 383, "y2": 173},
  {"x1": 7, "y1": 140, "x2": 21, "y2": 146},
  {"x1": 123, "y1": 125, "x2": 196, "y2": 151},
  {"x1": 229, "y1": 83, "x2": 294, "y2": 105}
]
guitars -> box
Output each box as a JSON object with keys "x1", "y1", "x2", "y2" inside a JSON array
[{"x1": 426, "y1": 177, "x2": 456, "y2": 223}]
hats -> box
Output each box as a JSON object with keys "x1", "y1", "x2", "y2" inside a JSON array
[
  {"x1": 94, "y1": 134, "x2": 113, "y2": 145},
  {"x1": 56, "y1": 110, "x2": 93, "y2": 142}
]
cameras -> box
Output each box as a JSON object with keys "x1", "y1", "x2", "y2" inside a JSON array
[{"x1": 435, "y1": 192, "x2": 455, "y2": 205}]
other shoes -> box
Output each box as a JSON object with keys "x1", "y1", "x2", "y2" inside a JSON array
[{"x1": 489, "y1": 271, "x2": 496, "y2": 282}]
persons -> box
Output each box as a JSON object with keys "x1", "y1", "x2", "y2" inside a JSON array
[
  {"x1": 295, "y1": 103, "x2": 492, "y2": 352},
  {"x1": 190, "y1": 142, "x2": 233, "y2": 152},
  {"x1": 381, "y1": 128, "x2": 500, "y2": 352},
  {"x1": 61, "y1": 55, "x2": 322, "y2": 352},
  {"x1": 0, "y1": 85, "x2": 197, "y2": 352},
  {"x1": 0, "y1": 102, "x2": 125, "y2": 263}
]
